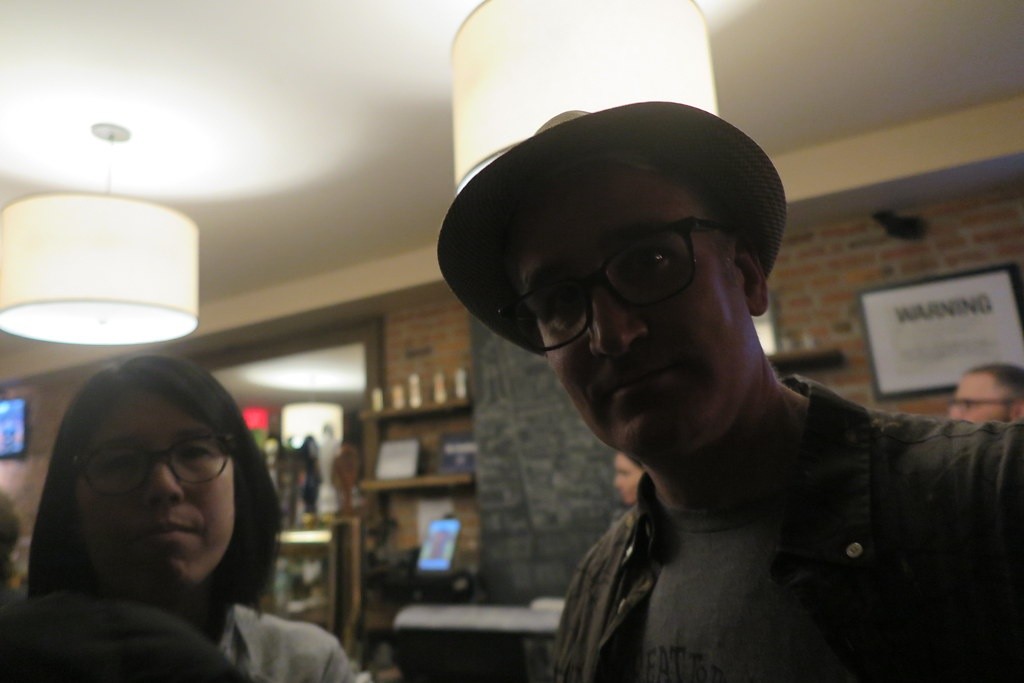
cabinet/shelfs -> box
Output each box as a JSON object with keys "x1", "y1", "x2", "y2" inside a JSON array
[{"x1": 359, "y1": 397, "x2": 474, "y2": 495}]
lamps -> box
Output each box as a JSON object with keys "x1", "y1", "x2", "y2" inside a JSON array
[
  {"x1": 281, "y1": 401, "x2": 346, "y2": 448},
  {"x1": 0, "y1": 122, "x2": 199, "y2": 346},
  {"x1": 451, "y1": 0, "x2": 718, "y2": 198}
]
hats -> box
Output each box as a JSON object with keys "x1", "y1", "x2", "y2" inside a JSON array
[{"x1": 437, "y1": 102, "x2": 787, "y2": 355}]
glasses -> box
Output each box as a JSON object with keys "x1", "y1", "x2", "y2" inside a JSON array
[
  {"x1": 73, "y1": 434, "x2": 229, "y2": 496},
  {"x1": 947, "y1": 399, "x2": 1011, "y2": 414},
  {"x1": 498, "y1": 216, "x2": 734, "y2": 352}
]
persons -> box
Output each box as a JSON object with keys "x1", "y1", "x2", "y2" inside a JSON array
[
  {"x1": 0, "y1": 490, "x2": 22, "y2": 606},
  {"x1": 608, "y1": 452, "x2": 643, "y2": 525},
  {"x1": 27, "y1": 357, "x2": 356, "y2": 683},
  {"x1": 950, "y1": 365, "x2": 1024, "y2": 423},
  {"x1": 437, "y1": 101, "x2": 1024, "y2": 683}
]
